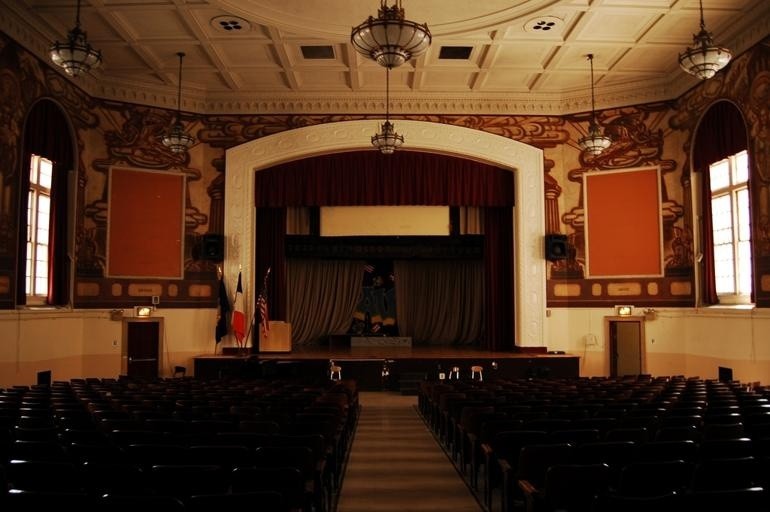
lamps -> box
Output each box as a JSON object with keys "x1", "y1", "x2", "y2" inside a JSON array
[
  {"x1": 577, "y1": 54, "x2": 613, "y2": 157},
  {"x1": 48, "y1": 0, "x2": 104, "y2": 79},
  {"x1": 677, "y1": 1, "x2": 733, "y2": 81},
  {"x1": 162, "y1": 53, "x2": 195, "y2": 154},
  {"x1": 350, "y1": 0, "x2": 432, "y2": 156}
]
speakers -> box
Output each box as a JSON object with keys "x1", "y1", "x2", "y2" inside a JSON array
[
  {"x1": 201, "y1": 231, "x2": 224, "y2": 262},
  {"x1": 546, "y1": 233, "x2": 569, "y2": 261}
]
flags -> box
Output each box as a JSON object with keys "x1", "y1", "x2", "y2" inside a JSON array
[
  {"x1": 257, "y1": 271, "x2": 269, "y2": 339},
  {"x1": 230, "y1": 271, "x2": 246, "y2": 346},
  {"x1": 215, "y1": 277, "x2": 233, "y2": 345}
]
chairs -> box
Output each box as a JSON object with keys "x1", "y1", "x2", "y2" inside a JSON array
[
  {"x1": 0, "y1": 374, "x2": 360, "y2": 512},
  {"x1": 417, "y1": 373, "x2": 770, "y2": 511}
]
